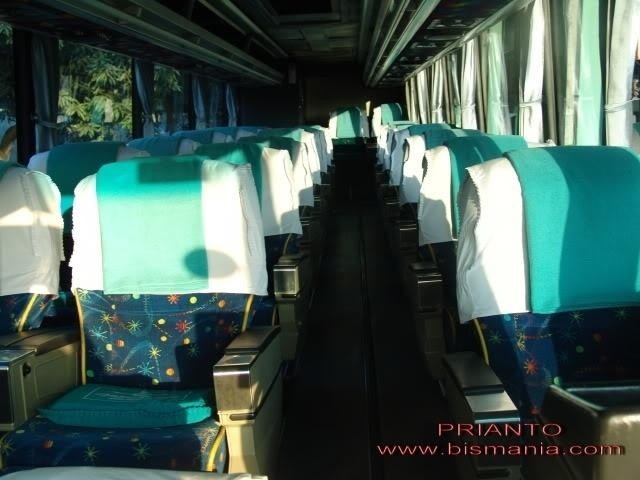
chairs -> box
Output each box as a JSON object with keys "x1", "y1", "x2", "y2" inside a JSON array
[
  {"x1": 370, "y1": 101, "x2": 638, "y2": 480},
  {"x1": 0, "y1": 124, "x2": 335, "y2": 477},
  {"x1": 327, "y1": 105, "x2": 370, "y2": 160}
]
